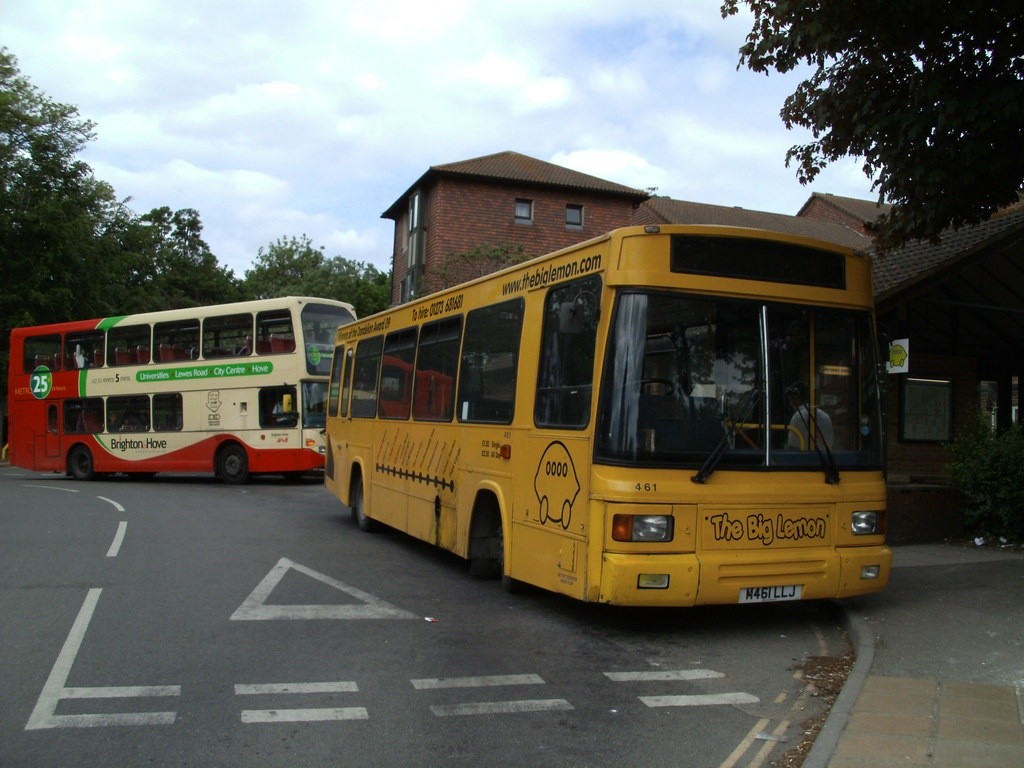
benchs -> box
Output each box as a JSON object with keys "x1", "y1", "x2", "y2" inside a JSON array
[{"x1": 35, "y1": 334, "x2": 295, "y2": 370}]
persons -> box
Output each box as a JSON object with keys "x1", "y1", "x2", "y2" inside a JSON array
[
  {"x1": 785, "y1": 381, "x2": 834, "y2": 452},
  {"x1": 118, "y1": 416, "x2": 135, "y2": 432},
  {"x1": 102, "y1": 411, "x2": 121, "y2": 433},
  {"x1": 75, "y1": 343, "x2": 90, "y2": 369},
  {"x1": 270, "y1": 389, "x2": 296, "y2": 424}
]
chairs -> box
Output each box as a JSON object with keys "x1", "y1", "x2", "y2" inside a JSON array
[{"x1": 75, "y1": 409, "x2": 101, "y2": 432}]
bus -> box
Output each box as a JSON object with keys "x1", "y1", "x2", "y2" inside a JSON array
[
  {"x1": 321, "y1": 224, "x2": 888, "y2": 607},
  {"x1": 7, "y1": 297, "x2": 359, "y2": 485}
]
